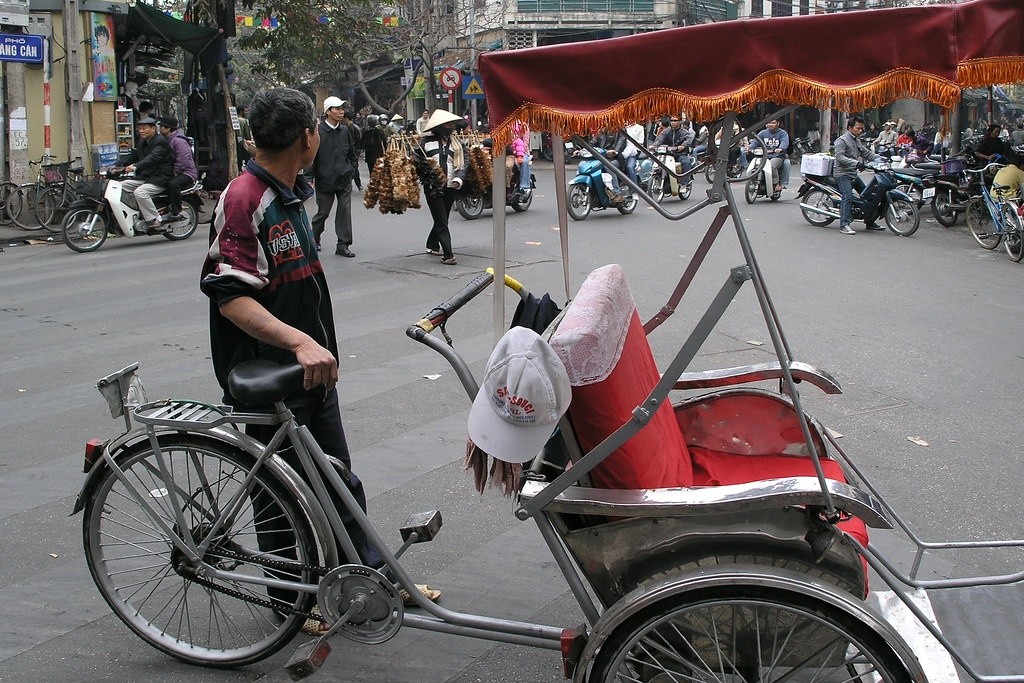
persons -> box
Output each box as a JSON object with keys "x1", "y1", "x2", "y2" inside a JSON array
[
  {"x1": 199, "y1": 86, "x2": 444, "y2": 638},
  {"x1": 301, "y1": 96, "x2": 357, "y2": 258},
  {"x1": 157, "y1": 115, "x2": 198, "y2": 221},
  {"x1": 149, "y1": 112, "x2": 531, "y2": 196},
  {"x1": 93, "y1": 25, "x2": 115, "y2": 58},
  {"x1": 119, "y1": 81, "x2": 145, "y2": 149},
  {"x1": 989, "y1": 154, "x2": 1024, "y2": 242},
  {"x1": 411, "y1": 108, "x2": 472, "y2": 265},
  {"x1": 544, "y1": 112, "x2": 1024, "y2": 219},
  {"x1": 138, "y1": 100, "x2": 154, "y2": 120},
  {"x1": 832, "y1": 116, "x2": 888, "y2": 235},
  {"x1": 106, "y1": 116, "x2": 177, "y2": 228}
]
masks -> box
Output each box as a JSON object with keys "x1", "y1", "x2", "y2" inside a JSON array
[{"x1": 380, "y1": 120, "x2": 388, "y2": 125}]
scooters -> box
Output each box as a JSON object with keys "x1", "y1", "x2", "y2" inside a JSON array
[
  {"x1": 538, "y1": 131, "x2": 573, "y2": 164},
  {"x1": 689, "y1": 136, "x2": 753, "y2": 185},
  {"x1": 788, "y1": 128, "x2": 989, "y2": 229}
]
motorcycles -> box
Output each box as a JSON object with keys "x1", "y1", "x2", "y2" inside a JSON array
[
  {"x1": 634, "y1": 148, "x2": 654, "y2": 192},
  {"x1": 794, "y1": 152, "x2": 920, "y2": 238},
  {"x1": 566, "y1": 135, "x2": 638, "y2": 221},
  {"x1": 744, "y1": 145, "x2": 783, "y2": 204},
  {"x1": 455, "y1": 138, "x2": 536, "y2": 220},
  {"x1": 647, "y1": 144, "x2": 696, "y2": 204},
  {"x1": 61, "y1": 164, "x2": 207, "y2": 252}
]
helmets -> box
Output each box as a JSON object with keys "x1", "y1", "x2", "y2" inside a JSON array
[{"x1": 379, "y1": 114, "x2": 389, "y2": 126}]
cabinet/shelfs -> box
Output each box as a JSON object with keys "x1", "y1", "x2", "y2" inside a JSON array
[{"x1": 116, "y1": 108, "x2": 134, "y2": 155}]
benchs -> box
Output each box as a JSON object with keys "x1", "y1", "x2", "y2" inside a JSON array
[{"x1": 547, "y1": 263, "x2": 868, "y2": 601}]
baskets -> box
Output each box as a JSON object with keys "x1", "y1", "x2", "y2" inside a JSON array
[
  {"x1": 75, "y1": 180, "x2": 100, "y2": 196},
  {"x1": 43, "y1": 165, "x2": 67, "y2": 183}
]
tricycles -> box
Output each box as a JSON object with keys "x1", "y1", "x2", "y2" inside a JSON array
[{"x1": 68, "y1": 1, "x2": 1024, "y2": 683}]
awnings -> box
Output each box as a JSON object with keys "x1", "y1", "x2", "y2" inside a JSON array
[
  {"x1": 121, "y1": 1, "x2": 228, "y2": 75},
  {"x1": 347, "y1": 63, "x2": 400, "y2": 85},
  {"x1": 413, "y1": 38, "x2": 503, "y2": 73}
]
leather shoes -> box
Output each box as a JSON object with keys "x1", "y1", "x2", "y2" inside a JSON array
[
  {"x1": 317, "y1": 243, "x2": 321, "y2": 251},
  {"x1": 336, "y1": 249, "x2": 355, "y2": 257}
]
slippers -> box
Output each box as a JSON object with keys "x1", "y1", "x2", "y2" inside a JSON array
[
  {"x1": 299, "y1": 604, "x2": 332, "y2": 635},
  {"x1": 392, "y1": 582, "x2": 442, "y2": 604}
]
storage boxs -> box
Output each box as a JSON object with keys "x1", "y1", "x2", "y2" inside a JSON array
[{"x1": 801, "y1": 154, "x2": 834, "y2": 176}]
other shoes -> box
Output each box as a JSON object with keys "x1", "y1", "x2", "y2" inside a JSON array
[
  {"x1": 441, "y1": 256, "x2": 457, "y2": 265},
  {"x1": 148, "y1": 219, "x2": 162, "y2": 228},
  {"x1": 516, "y1": 164, "x2": 885, "y2": 235},
  {"x1": 172, "y1": 208, "x2": 182, "y2": 217},
  {"x1": 426, "y1": 248, "x2": 444, "y2": 255}
]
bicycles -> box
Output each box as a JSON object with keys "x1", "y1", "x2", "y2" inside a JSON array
[
  {"x1": 962, "y1": 162, "x2": 1024, "y2": 262},
  {"x1": 0, "y1": 177, "x2": 24, "y2": 226},
  {"x1": 5, "y1": 153, "x2": 108, "y2": 233}
]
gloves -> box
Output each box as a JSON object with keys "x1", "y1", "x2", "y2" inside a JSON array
[
  {"x1": 881, "y1": 158, "x2": 894, "y2": 164},
  {"x1": 856, "y1": 162, "x2": 866, "y2": 172}
]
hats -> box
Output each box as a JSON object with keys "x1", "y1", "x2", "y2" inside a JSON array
[
  {"x1": 890, "y1": 121, "x2": 897, "y2": 125},
  {"x1": 391, "y1": 114, "x2": 404, "y2": 121},
  {"x1": 157, "y1": 116, "x2": 177, "y2": 131},
  {"x1": 885, "y1": 121, "x2": 891, "y2": 126},
  {"x1": 323, "y1": 96, "x2": 348, "y2": 114},
  {"x1": 467, "y1": 325, "x2": 573, "y2": 464},
  {"x1": 422, "y1": 109, "x2": 463, "y2": 133},
  {"x1": 137, "y1": 118, "x2": 156, "y2": 124}
]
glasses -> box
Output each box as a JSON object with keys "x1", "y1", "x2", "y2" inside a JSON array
[{"x1": 670, "y1": 120, "x2": 680, "y2": 122}]
